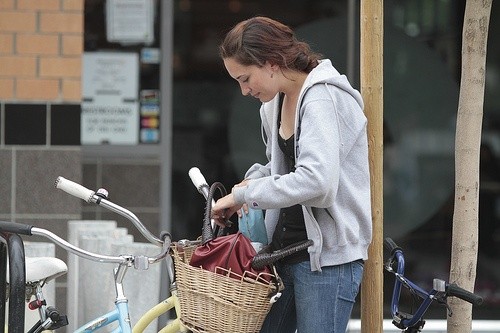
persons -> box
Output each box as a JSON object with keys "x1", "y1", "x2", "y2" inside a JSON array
[{"x1": 210, "y1": 15, "x2": 372, "y2": 333}]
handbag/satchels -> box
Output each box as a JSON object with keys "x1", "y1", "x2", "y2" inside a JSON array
[{"x1": 190, "y1": 182, "x2": 314, "y2": 285}]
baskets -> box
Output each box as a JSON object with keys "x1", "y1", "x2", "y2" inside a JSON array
[{"x1": 172, "y1": 239, "x2": 285, "y2": 333}]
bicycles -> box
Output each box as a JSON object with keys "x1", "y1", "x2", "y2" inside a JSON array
[
  {"x1": 55, "y1": 167, "x2": 285, "y2": 333},
  {"x1": 383, "y1": 237, "x2": 483, "y2": 333},
  {"x1": 0, "y1": 221, "x2": 171, "y2": 333}
]
